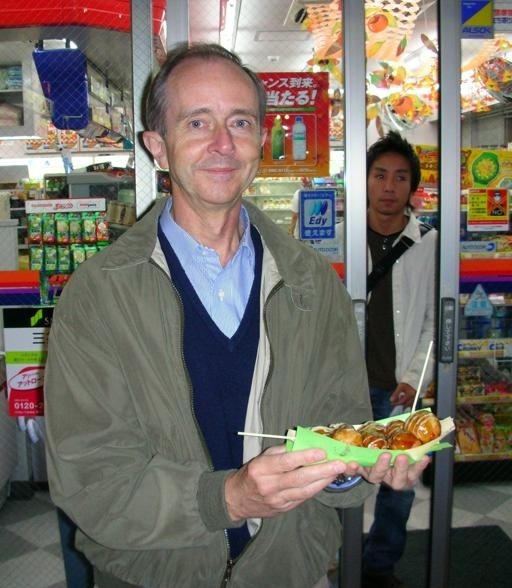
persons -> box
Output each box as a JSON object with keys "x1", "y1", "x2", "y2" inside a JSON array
[
  {"x1": 290, "y1": 176, "x2": 311, "y2": 239},
  {"x1": 43, "y1": 44, "x2": 431, "y2": 588},
  {"x1": 319, "y1": 131, "x2": 437, "y2": 588}
]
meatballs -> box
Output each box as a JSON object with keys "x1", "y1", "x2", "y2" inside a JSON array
[{"x1": 314, "y1": 409, "x2": 442, "y2": 449}]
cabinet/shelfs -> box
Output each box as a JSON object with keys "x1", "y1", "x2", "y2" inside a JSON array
[
  {"x1": 455, "y1": 237, "x2": 512, "y2": 465},
  {"x1": 1, "y1": 1, "x2": 160, "y2": 501}
]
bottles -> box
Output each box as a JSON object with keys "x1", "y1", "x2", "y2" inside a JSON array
[{"x1": 271, "y1": 115, "x2": 308, "y2": 161}]
